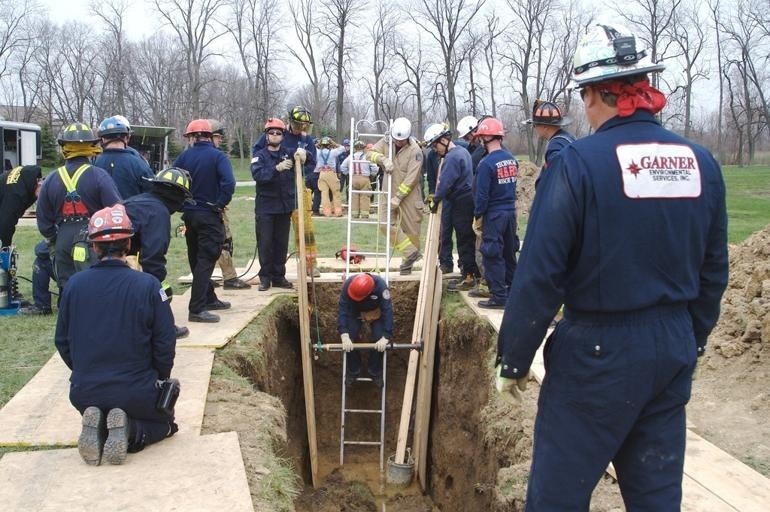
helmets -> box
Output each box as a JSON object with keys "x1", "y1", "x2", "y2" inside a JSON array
[
  {"x1": 58, "y1": 123, "x2": 101, "y2": 145},
  {"x1": 142, "y1": 167, "x2": 195, "y2": 201},
  {"x1": 392, "y1": 118, "x2": 412, "y2": 141},
  {"x1": 335, "y1": 245, "x2": 366, "y2": 263},
  {"x1": 521, "y1": 101, "x2": 574, "y2": 128},
  {"x1": 425, "y1": 117, "x2": 505, "y2": 148},
  {"x1": 97, "y1": 118, "x2": 129, "y2": 139},
  {"x1": 183, "y1": 119, "x2": 225, "y2": 139},
  {"x1": 313, "y1": 135, "x2": 374, "y2": 150},
  {"x1": 85, "y1": 207, "x2": 136, "y2": 244},
  {"x1": 564, "y1": 23, "x2": 668, "y2": 92},
  {"x1": 114, "y1": 115, "x2": 130, "y2": 136},
  {"x1": 348, "y1": 274, "x2": 376, "y2": 303},
  {"x1": 265, "y1": 118, "x2": 287, "y2": 131},
  {"x1": 290, "y1": 106, "x2": 313, "y2": 125}
]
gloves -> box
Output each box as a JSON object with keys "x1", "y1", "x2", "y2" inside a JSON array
[
  {"x1": 276, "y1": 159, "x2": 293, "y2": 172},
  {"x1": 207, "y1": 202, "x2": 226, "y2": 214},
  {"x1": 381, "y1": 157, "x2": 395, "y2": 174},
  {"x1": 472, "y1": 216, "x2": 484, "y2": 237},
  {"x1": 375, "y1": 337, "x2": 389, "y2": 353},
  {"x1": 294, "y1": 148, "x2": 306, "y2": 164},
  {"x1": 429, "y1": 200, "x2": 439, "y2": 214},
  {"x1": 341, "y1": 333, "x2": 354, "y2": 352},
  {"x1": 46, "y1": 233, "x2": 57, "y2": 260},
  {"x1": 496, "y1": 363, "x2": 529, "y2": 410},
  {"x1": 391, "y1": 197, "x2": 402, "y2": 212}
]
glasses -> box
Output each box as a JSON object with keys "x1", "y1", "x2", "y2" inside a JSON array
[
  {"x1": 581, "y1": 89, "x2": 587, "y2": 102},
  {"x1": 268, "y1": 131, "x2": 281, "y2": 135}
]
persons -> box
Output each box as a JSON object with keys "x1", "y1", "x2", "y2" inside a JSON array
[
  {"x1": 366, "y1": 117, "x2": 423, "y2": 276},
  {"x1": 250, "y1": 118, "x2": 306, "y2": 291},
  {"x1": 52, "y1": 202, "x2": 179, "y2": 469},
  {"x1": 250, "y1": 106, "x2": 321, "y2": 276},
  {"x1": 306, "y1": 136, "x2": 380, "y2": 218},
  {"x1": 73, "y1": 167, "x2": 192, "y2": 337},
  {"x1": 336, "y1": 270, "x2": 398, "y2": 390},
  {"x1": 174, "y1": 119, "x2": 251, "y2": 321},
  {"x1": 524, "y1": 98, "x2": 578, "y2": 191},
  {"x1": 1, "y1": 165, "x2": 43, "y2": 306},
  {"x1": 492, "y1": 23, "x2": 731, "y2": 511},
  {"x1": 423, "y1": 122, "x2": 477, "y2": 291},
  {"x1": 95, "y1": 115, "x2": 154, "y2": 199},
  {"x1": 457, "y1": 114, "x2": 520, "y2": 306},
  {"x1": 16, "y1": 121, "x2": 121, "y2": 315}
]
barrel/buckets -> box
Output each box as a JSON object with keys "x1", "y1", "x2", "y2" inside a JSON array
[{"x1": 386, "y1": 448, "x2": 419, "y2": 488}]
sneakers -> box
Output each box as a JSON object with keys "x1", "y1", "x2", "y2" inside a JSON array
[
  {"x1": 272, "y1": 279, "x2": 292, "y2": 288},
  {"x1": 439, "y1": 265, "x2": 453, "y2": 273},
  {"x1": 346, "y1": 375, "x2": 361, "y2": 386},
  {"x1": 175, "y1": 326, "x2": 189, "y2": 339},
  {"x1": 259, "y1": 280, "x2": 270, "y2": 290},
  {"x1": 189, "y1": 310, "x2": 220, "y2": 322},
  {"x1": 308, "y1": 268, "x2": 321, "y2": 277},
  {"x1": 446, "y1": 273, "x2": 493, "y2": 298},
  {"x1": 368, "y1": 372, "x2": 384, "y2": 389},
  {"x1": 78, "y1": 406, "x2": 108, "y2": 466},
  {"x1": 400, "y1": 267, "x2": 411, "y2": 275},
  {"x1": 17, "y1": 305, "x2": 52, "y2": 316},
  {"x1": 103, "y1": 408, "x2": 130, "y2": 467},
  {"x1": 224, "y1": 278, "x2": 251, "y2": 289},
  {"x1": 478, "y1": 296, "x2": 507, "y2": 308},
  {"x1": 207, "y1": 298, "x2": 231, "y2": 310}
]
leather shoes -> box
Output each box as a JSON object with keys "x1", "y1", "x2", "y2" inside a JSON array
[{"x1": 400, "y1": 251, "x2": 424, "y2": 271}]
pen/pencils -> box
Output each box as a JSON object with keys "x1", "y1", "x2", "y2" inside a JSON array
[{"x1": 137, "y1": 251, "x2": 139, "y2": 270}]
